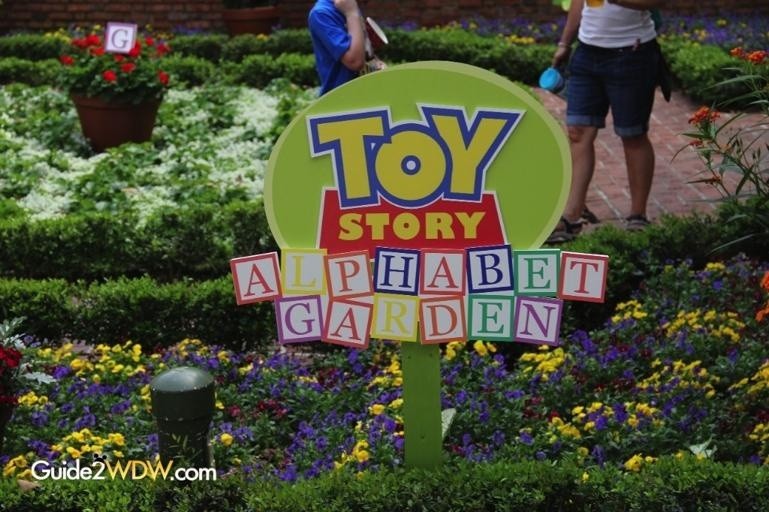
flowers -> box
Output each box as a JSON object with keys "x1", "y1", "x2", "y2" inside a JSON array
[
  {"x1": 668, "y1": 46, "x2": 769, "y2": 324},
  {"x1": 0, "y1": 345, "x2": 24, "y2": 428},
  {"x1": 60, "y1": 35, "x2": 173, "y2": 106}
]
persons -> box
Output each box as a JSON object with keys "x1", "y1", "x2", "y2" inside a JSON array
[
  {"x1": 542, "y1": 0, "x2": 674, "y2": 244},
  {"x1": 308, "y1": 0, "x2": 380, "y2": 99}
]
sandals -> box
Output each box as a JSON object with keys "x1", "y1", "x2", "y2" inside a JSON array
[
  {"x1": 546, "y1": 216, "x2": 586, "y2": 241},
  {"x1": 627, "y1": 214, "x2": 651, "y2": 233}
]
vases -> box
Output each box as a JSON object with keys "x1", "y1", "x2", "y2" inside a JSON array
[{"x1": 71, "y1": 91, "x2": 164, "y2": 152}]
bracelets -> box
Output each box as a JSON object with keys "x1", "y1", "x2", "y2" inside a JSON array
[{"x1": 557, "y1": 42, "x2": 573, "y2": 50}]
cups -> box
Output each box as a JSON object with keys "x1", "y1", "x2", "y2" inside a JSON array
[{"x1": 540, "y1": 69, "x2": 569, "y2": 100}]
